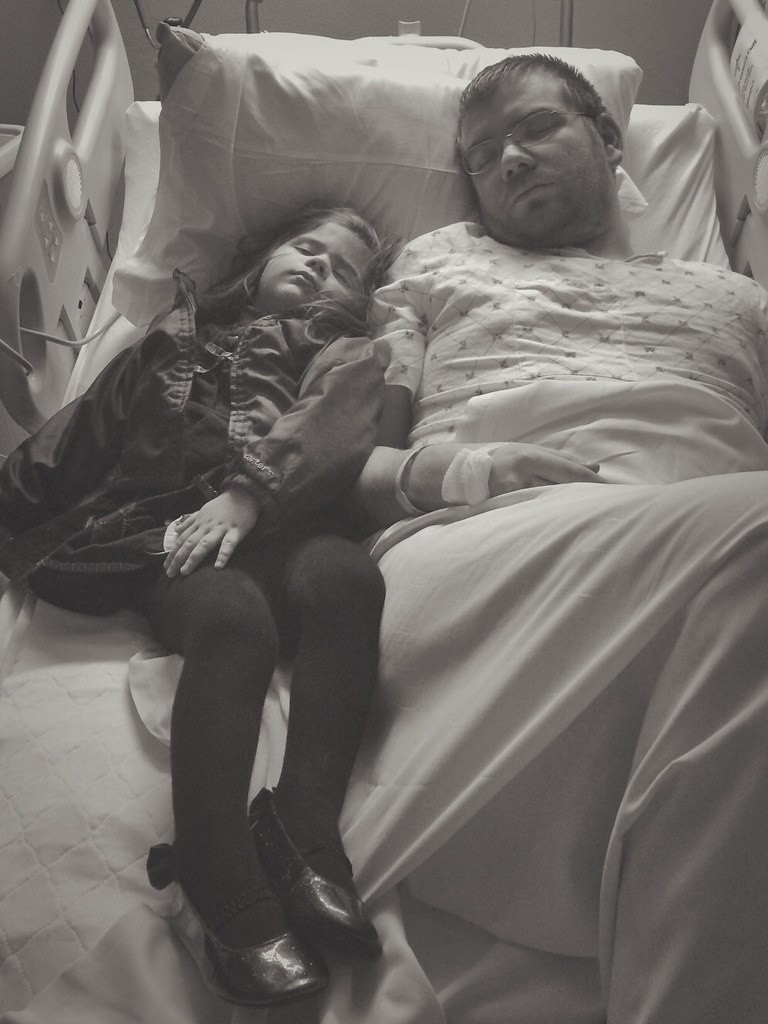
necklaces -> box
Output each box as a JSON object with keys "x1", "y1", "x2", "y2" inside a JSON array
[{"x1": 394, "y1": 443, "x2": 431, "y2": 517}]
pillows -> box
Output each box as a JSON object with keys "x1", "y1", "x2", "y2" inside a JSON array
[
  {"x1": 261, "y1": 31, "x2": 644, "y2": 174},
  {"x1": 111, "y1": 20, "x2": 649, "y2": 333}
]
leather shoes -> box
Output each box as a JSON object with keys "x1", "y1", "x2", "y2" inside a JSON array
[
  {"x1": 248, "y1": 787, "x2": 386, "y2": 958},
  {"x1": 146, "y1": 844, "x2": 332, "y2": 1007}
]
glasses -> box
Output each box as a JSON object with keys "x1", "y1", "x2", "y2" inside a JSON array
[{"x1": 461, "y1": 111, "x2": 595, "y2": 175}]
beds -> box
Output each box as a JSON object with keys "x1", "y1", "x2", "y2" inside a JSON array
[{"x1": 0, "y1": 0, "x2": 768, "y2": 1024}]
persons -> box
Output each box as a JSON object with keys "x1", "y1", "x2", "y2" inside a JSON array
[
  {"x1": 0, "y1": 206, "x2": 386, "y2": 1004},
  {"x1": 352, "y1": 55, "x2": 768, "y2": 1023}
]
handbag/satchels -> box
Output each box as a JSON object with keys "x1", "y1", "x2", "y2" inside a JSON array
[{"x1": 0, "y1": 493, "x2": 208, "y2": 616}]
